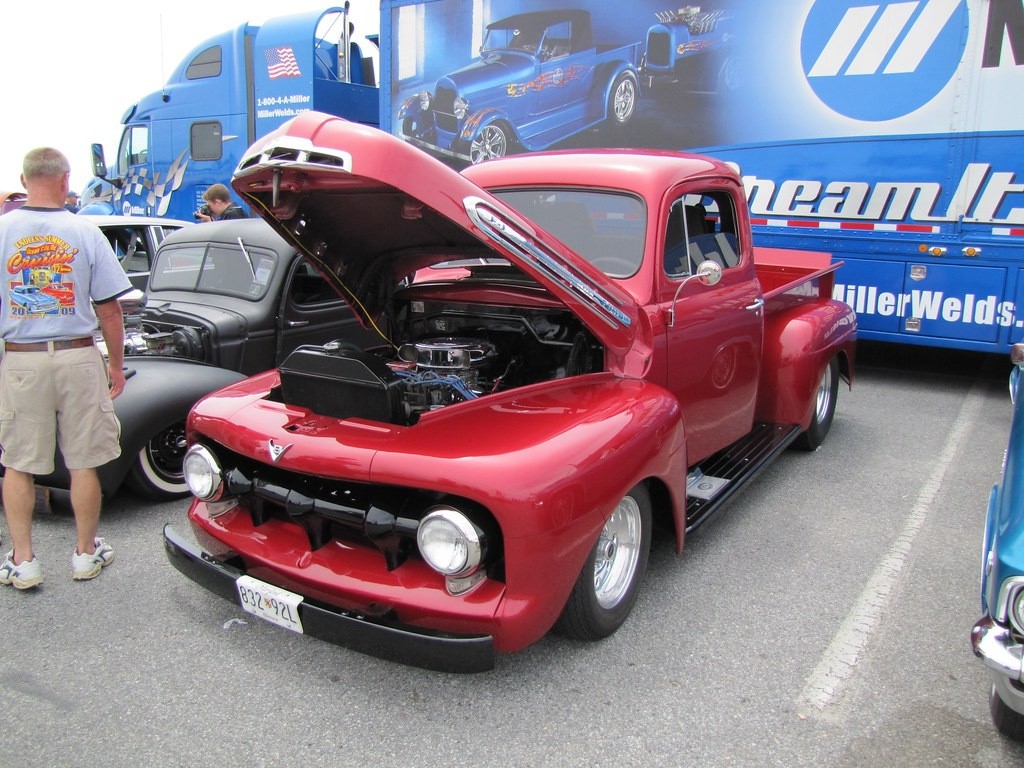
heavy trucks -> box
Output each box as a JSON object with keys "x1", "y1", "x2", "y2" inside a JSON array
[{"x1": 76, "y1": 1, "x2": 1024, "y2": 356}]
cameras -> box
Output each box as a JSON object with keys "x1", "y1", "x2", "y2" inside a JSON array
[{"x1": 195, "y1": 206, "x2": 212, "y2": 219}]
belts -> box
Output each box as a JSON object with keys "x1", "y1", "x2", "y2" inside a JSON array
[{"x1": 5, "y1": 336, "x2": 94, "y2": 351}]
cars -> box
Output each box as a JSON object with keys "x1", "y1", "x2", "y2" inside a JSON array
[
  {"x1": 971, "y1": 338, "x2": 1024, "y2": 741},
  {"x1": 85, "y1": 216, "x2": 217, "y2": 301},
  {"x1": 35, "y1": 219, "x2": 396, "y2": 504}
]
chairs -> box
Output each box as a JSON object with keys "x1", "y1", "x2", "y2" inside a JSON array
[
  {"x1": 542, "y1": 203, "x2": 594, "y2": 262},
  {"x1": 634, "y1": 209, "x2": 712, "y2": 272}
]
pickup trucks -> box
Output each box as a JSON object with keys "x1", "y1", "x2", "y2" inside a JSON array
[
  {"x1": 164, "y1": 110, "x2": 858, "y2": 673},
  {"x1": 395, "y1": 1, "x2": 643, "y2": 167},
  {"x1": 644, "y1": 4, "x2": 761, "y2": 101}
]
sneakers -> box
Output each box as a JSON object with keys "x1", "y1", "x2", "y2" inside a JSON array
[
  {"x1": 71, "y1": 536, "x2": 115, "y2": 580},
  {"x1": 0, "y1": 549, "x2": 44, "y2": 590}
]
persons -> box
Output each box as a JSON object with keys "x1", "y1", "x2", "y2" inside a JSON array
[
  {"x1": 196, "y1": 182, "x2": 248, "y2": 222},
  {"x1": 0, "y1": 146, "x2": 135, "y2": 591}
]
